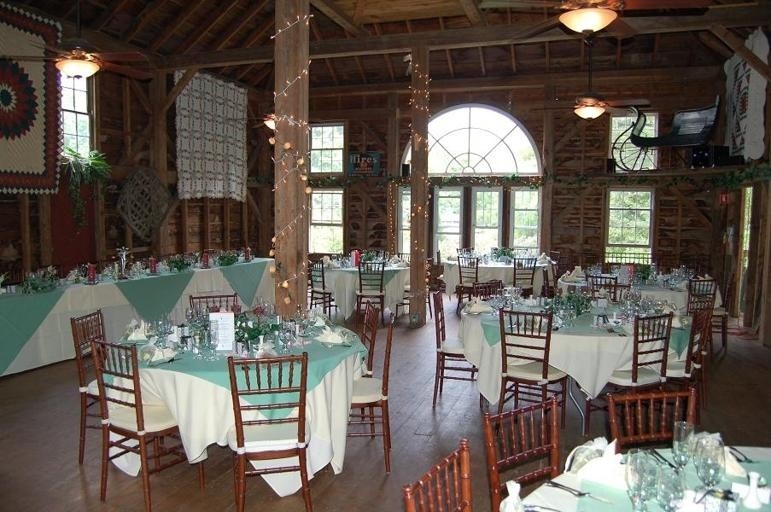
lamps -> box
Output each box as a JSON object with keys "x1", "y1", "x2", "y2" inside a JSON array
[
  {"x1": 573, "y1": 100, "x2": 607, "y2": 122},
  {"x1": 54, "y1": 45, "x2": 103, "y2": 81},
  {"x1": 554, "y1": 7, "x2": 619, "y2": 39}
]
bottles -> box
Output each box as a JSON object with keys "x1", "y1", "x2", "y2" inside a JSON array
[
  {"x1": 552, "y1": 290, "x2": 591, "y2": 329},
  {"x1": 742, "y1": 472, "x2": 762, "y2": 509},
  {"x1": 351, "y1": 249, "x2": 360, "y2": 266}
]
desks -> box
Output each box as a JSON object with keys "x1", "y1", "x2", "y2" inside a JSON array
[{"x1": 518, "y1": 446, "x2": 770, "y2": 511}]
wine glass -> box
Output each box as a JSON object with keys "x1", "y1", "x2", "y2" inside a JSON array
[
  {"x1": 141, "y1": 305, "x2": 220, "y2": 362},
  {"x1": 626, "y1": 421, "x2": 726, "y2": 511},
  {"x1": 488, "y1": 285, "x2": 523, "y2": 317},
  {"x1": 270, "y1": 309, "x2": 317, "y2": 355},
  {"x1": 623, "y1": 295, "x2": 678, "y2": 327}
]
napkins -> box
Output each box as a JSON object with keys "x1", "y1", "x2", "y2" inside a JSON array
[{"x1": 499, "y1": 479, "x2": 524, "y2": 511}]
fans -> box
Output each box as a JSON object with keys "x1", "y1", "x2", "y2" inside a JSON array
[
  {"x1": 226, "y1": 112, "x2": 323, "y2": 129},
  {"x1": 481, "y1": 0, "x2": 708, "y2": 44},
  {"x1": 530, "y1": 46, "x2": 652, "y2": 126},
  {"x1": 0, "y1": 0, "x2": 155, "y2": 83}
]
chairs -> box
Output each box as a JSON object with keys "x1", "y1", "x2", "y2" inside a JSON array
[
  {"x1": 607, "y1": 386, "x2": 696, "y2": 456},
  {"x1": 425, "y1": 244, "x2": 730, "y2": 437},
  {"x1": 486, "y1": 394, "x2": 559, "y2": 512},
  {"x1": 69, "y1": 243, "x2": 411, "y2": 511},
  {"x1": 611, "y1": 91, "x2": 721, "y2": 172},
  {"x1": 403, "y1": 440, "x2": 472, "y2": 512}
]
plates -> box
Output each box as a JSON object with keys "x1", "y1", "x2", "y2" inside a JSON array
[{"x1": 759, "y1": 474, "x2": 768, "y2": 486}]
